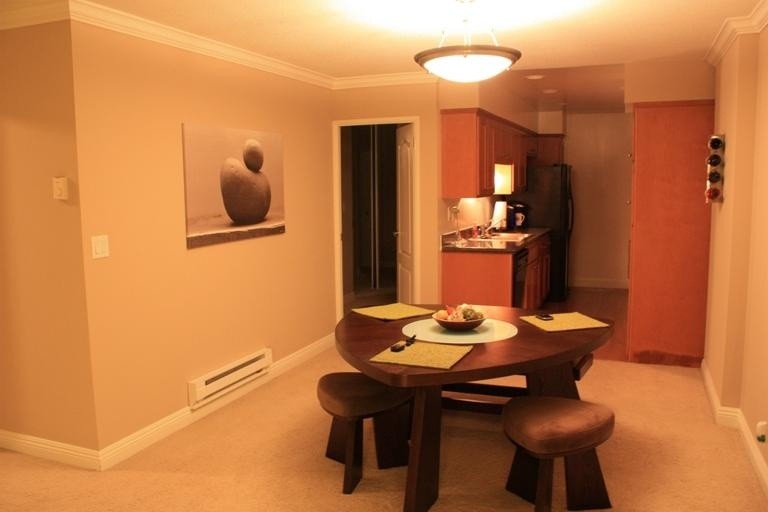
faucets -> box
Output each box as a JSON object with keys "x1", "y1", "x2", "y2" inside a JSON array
[{"x1": 481, "y1": 217, "x2": 506, "y2": 237}]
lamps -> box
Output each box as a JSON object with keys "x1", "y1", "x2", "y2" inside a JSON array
[{"x1": 415, "y1": 44, "x2": 522, "y2": 84}]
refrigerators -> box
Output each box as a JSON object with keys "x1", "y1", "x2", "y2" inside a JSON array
[{"x1": 505, "y1": 164, "x2": 574, "y2": 302}]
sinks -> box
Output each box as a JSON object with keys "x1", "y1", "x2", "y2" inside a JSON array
[
  {"x1": 468, "y1": 237, "x2": 524, "y2": 242},
  {"x1": 467, "y1": 232, "x2": 535, "y2": 237}
]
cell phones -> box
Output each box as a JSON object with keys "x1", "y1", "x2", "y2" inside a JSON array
[{"x1": 536, "y1": 311, "x2": 554, "y2": 321}]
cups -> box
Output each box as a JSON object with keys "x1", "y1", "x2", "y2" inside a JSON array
[{"x1": 516, "y1": 213, "x2": 524, "y2": 225}]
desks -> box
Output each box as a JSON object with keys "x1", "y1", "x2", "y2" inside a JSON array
[{"x1": 335, "y1": 292, "x2": 614, "y2": 512}]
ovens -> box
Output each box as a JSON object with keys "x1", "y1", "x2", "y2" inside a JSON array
[{"x1": 512, "y1": 254, "x2": 528, "y2": 308}]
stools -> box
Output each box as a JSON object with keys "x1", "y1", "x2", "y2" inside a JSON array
[
  {"x1": 501, "y1": 393, "x2": 614, "y2": 512},
  {"x1": 315, "y1": 371, "x2": 414, "y2": 489}
]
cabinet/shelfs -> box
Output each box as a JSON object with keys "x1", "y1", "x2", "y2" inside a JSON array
[
  {"x1": 440, "y1": 110, "x2": 540, "y2": 198},
  {"x1": 441, "y1": 234, "x2": 552, "y2": 323}
]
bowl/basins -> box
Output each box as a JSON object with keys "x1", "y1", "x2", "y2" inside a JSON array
[{"x1": 433, "y1": 310, "x2": 488, "y2": 332}]
